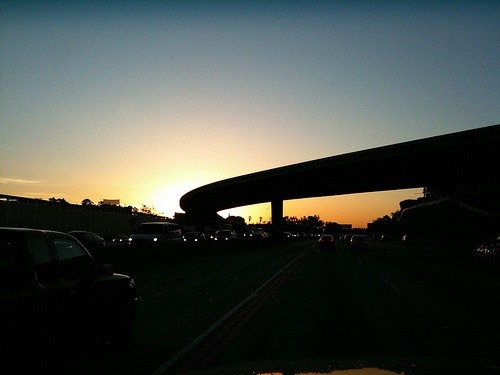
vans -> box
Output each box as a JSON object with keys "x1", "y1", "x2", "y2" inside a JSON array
[{"x1": 128, "y1": 222, "x2": 182, "y2": 253}]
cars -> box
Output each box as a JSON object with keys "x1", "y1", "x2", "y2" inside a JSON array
[
  {"x1": 112, "y1": 235, "x2": 129, "y2": 247},
  {"x1": 244, "y1": 231, "x2": 308, "y2": 242},
  {"x1": 184, "y1": 232, "x2": 206, "y2": 247},
  {"x1": 70, "y1": 230, "x2": 104, "y2": 250},
  {"x1": 340, "y1": 234, "x2": 368, "y2": 246},
  {"x1": 213, "y1": 229, "x2": 236, "y2": 241},
  {"x1": 319, "y1": 234, "x2": 336, "y2": 249}
]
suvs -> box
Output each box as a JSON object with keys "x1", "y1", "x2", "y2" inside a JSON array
[{"x1": 1, "y1": 225, "x2": 137, "y2": 371}]
